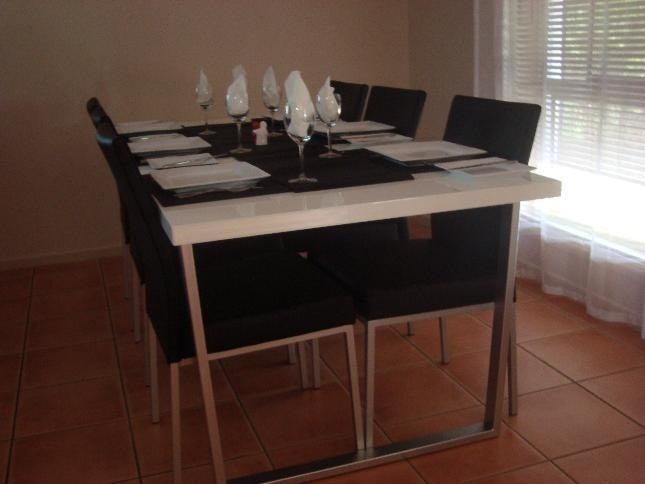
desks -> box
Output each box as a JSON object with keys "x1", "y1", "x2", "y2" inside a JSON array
[{"x1": 113, "y1": 107, "x2": 561, "y2": 484}]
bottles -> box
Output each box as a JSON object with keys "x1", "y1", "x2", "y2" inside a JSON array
[{"x1": 252, "y1": 120, "x2": 268, "y2": 145}]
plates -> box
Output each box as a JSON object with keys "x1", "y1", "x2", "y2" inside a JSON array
[
  {"x1": 313, "y1": 118, "x2": 395, "y2": 135},
  {"x1": 127, "y1": 135, "x2": 212, "y2": 154},
  {"x1": 364, "y1": 140, "x2": 487, "y2": 166},
  {"x1": 150, "y1": 158, "x2": 271, "y2": 190}
]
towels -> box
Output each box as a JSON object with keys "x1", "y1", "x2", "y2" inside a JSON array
[
  {"x1": 196, "y1": 68, "x2": 213, "y2": 104},
  {"x1": 282, "y1": 70, "x2": 315, "y2": 137},
  {"x1": 261, "y1": 66, "x2": 280, "y2": 109},
  {"x1": 225, "y1": 65, "x2": 250, "y2": 114}
]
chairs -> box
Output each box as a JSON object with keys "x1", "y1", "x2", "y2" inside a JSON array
[
  {"x1": 323, "y1": 77, "x2": 429, "y2": 141},
  {"x1": 85, "y1": 95, "x2": 364, "y2": 483},
  {"x1": 362, "y1": 95, "x2": 541, "y2": 445}
]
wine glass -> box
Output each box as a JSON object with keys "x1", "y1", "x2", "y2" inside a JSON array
[
  {"x1": 316, "y1": 94, "x2": 342, "y2": 158},
  {"x1": 285, "y1": 99, "x2": 318, "y2": 182},
  {"x1": 191, "y1": 81, "x2": 217, "y2": 135},
  {"x1": 225, "y1": 93, "x2": 251, "y2": 153},
  {"x1": 260, "y1": 86, "x2": 283, "y2": 136}
]
